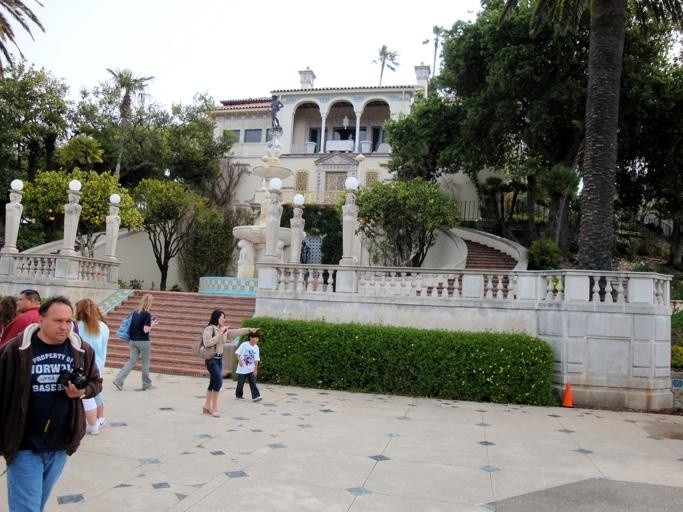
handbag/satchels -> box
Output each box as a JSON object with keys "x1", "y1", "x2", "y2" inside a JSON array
[
  {"x1": 116, "y1": 318, "x2": 132, "y2": 340},
  {"x1": 192, "y1": 344, "x2": 216, "y2": 359}
]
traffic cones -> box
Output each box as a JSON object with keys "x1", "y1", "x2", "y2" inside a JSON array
[{"x1": 560, "y1": 379, "x2": 574, "y2": 408}]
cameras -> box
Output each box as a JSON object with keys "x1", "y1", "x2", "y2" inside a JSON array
[{"x1": 58, "y1": 367, "x2": 90, "y2": 389}]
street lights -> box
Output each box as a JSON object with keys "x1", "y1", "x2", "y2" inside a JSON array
[
  {"x1": 99, "y1": 193, "x2": 121, "y2": 261},
  {"x1": 54, "y1": 179, "x2": 83, "y2": 256},
  {"x1": 287, "y1": 193, "x2": 305, "y2": 274},
  {"x1": 335, "y1": 175, "x2": 360, "y2": 266},
  {"x1": 1, "y1": 177, "x2": 25, "y2": 253},
  {"x1": 259, "y1": 176, "x2": 283, "y2": 263}
]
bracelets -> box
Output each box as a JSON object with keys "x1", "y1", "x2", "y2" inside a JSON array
[{"x1": 254, "y1": 368, "x2": 257, "y2": 370}]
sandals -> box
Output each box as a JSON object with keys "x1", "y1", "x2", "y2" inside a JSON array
[{"x1": 203, "y1": 406, "x2": 221, "y2": 417}]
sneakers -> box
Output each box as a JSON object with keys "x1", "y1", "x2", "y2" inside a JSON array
[
  {"x1": 113, "y1": 381, "x2": 122, "y2": 390},
  {"x1": 237, "y1": 396, "x2": 262, "y2": 401},
  {"x1": 143, "y1": 384, "x2": 154, "y2": 390},
  {"x1": 86, "y1": 417, "x2": 105, "y2": 434}
]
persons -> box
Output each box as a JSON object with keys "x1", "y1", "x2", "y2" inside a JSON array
[
  {"x1": 112, "y1": 294, "x2": 159, "y2": 391},
  {"x1": 235, "y1": 332, "x2": 262, "y2": 402},
  {"x1": 190, "y1": 310, "x2": 261, "y2": 417},
  {"x1": 0, "y1": 288, "x2": 108, "y2": 512}
]
